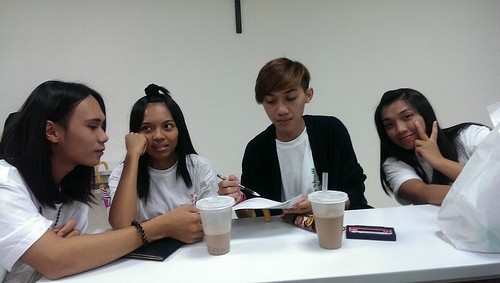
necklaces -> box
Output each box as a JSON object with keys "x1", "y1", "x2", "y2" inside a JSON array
[{"x1": 38, "y1": 207, "x2": 60, "y2": 227}]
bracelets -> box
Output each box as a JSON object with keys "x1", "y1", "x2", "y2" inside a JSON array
[{"x1": 130, "y1": 219, "x2": 149, "y2": 247}]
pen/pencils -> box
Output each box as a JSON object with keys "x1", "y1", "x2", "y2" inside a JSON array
[{"x1": 217, "y1": 175, "x2": 261, "y2": 196}]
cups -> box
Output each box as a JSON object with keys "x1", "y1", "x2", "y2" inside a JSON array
[
  {"x1": 195, "y1": 195, "x2": 235, "y2": 256},
  {"x1": 307, "y1": 189, "x2": 348, "y2": 250}
]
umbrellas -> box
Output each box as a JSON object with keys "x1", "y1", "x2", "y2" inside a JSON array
[{"x1": 95, "y1": 161, "x2": 111, "y2": 208}]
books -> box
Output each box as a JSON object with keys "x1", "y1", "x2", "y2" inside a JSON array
[{"x1": 125, "y1": 236, "x2": 184, "y2": 262}]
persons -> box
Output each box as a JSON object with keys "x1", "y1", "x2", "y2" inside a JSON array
[
  {"x1": 107, "y1": 83, "x2": 219, "y2": 231},
  {"x1": 217, "y1": 57, "x2": 374, "y2": 219},
  {"x1": 0, "y1": 80, "x2": 206, "y2": 283},
  {"x1": 374, "y1": 87, "x2": 492, "y2": 207}
]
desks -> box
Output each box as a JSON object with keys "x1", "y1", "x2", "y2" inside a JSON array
[{"x1": 40, "y1": 203, "x2": 500, "y2": 283}]
